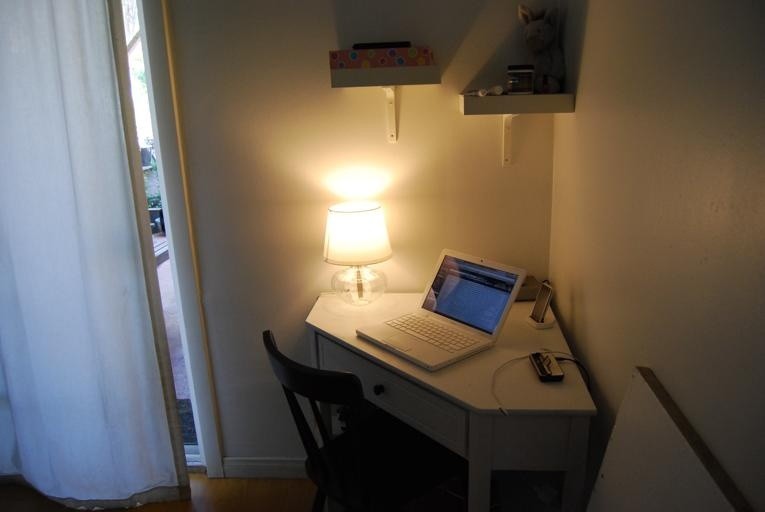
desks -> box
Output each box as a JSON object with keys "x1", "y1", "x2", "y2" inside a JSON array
[{"x1": 305, "y1": 290, "x2": 597, "y2": 511}]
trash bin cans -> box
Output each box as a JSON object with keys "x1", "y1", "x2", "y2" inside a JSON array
[{"x1": 154, "y1": 218, "x2": 162, "y2": 231}]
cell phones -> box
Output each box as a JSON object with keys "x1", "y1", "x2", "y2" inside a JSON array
[{"x1": 530, "y1": 283, "x2": 552, "y2": 322}]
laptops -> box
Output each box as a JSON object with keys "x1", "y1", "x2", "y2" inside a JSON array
[{"x1": 356, "y1": 248, "x2": 526, "y2": 373}]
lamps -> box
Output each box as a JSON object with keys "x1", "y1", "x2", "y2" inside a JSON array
[{"x1": 323, "y1": 199, "x2": 395, "y2": 306}]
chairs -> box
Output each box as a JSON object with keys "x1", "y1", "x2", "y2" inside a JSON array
[{"x1": 262, "y1": 329, "x2": 449, "y2": 512}]
